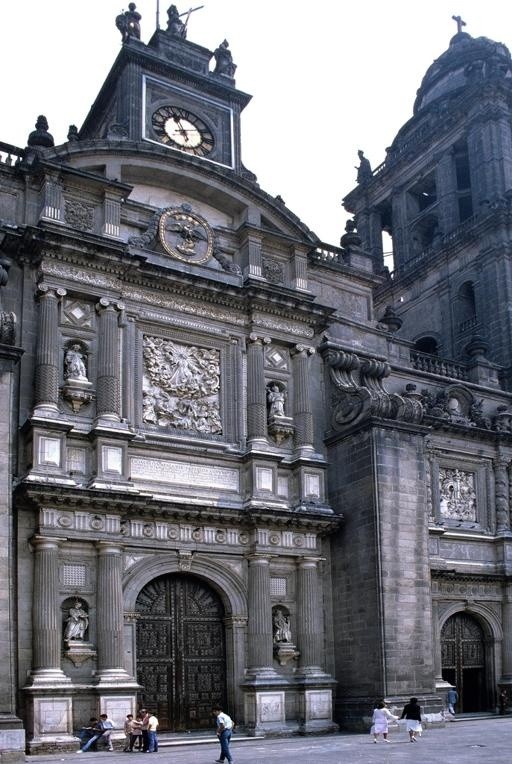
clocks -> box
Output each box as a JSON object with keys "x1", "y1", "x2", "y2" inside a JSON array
[{"x1": 150, "y1": 104, "x2": 215, "y2": 157}]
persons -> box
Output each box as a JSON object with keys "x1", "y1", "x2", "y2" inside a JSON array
[
  {"x1": 169, "y1": 352, "x2": 202, "y2": 384},
  {"x1": 273, "y1": 610, "x2": 292, "y2": 641},
  {"x1": 125, "y1": 1, "x2": 141, "y2": 38},
  {"x1": 143, "y1": 387, "x2": 224, "y2": 435},
  {"x1": 448, "y1": 688, "x2": 459, "y2": 715},
  {"x1": 75, "y1": 708, "x2": 159, "y2": 753},
  {"x1": 63, "y1": 602, "x2": 90, "y2": 640},
  {"x1": 212, "y1": 703, "x2": 236, "y2": 764},
  {"x1": 398, "y1": 697, "x2": 422, "y2": 742},
  {"x1": 267, "y1": 384, "x2": 285, "y2": 415},
  {"x1": 372, "y1": 701, "x2": 399, "y2": 743},
  {"x1": 354, "y1": 148, "x2": 372, "y2": 185},
  {"x1": 64, "y1": 343, "x2": 88, "y2": 380}
]
